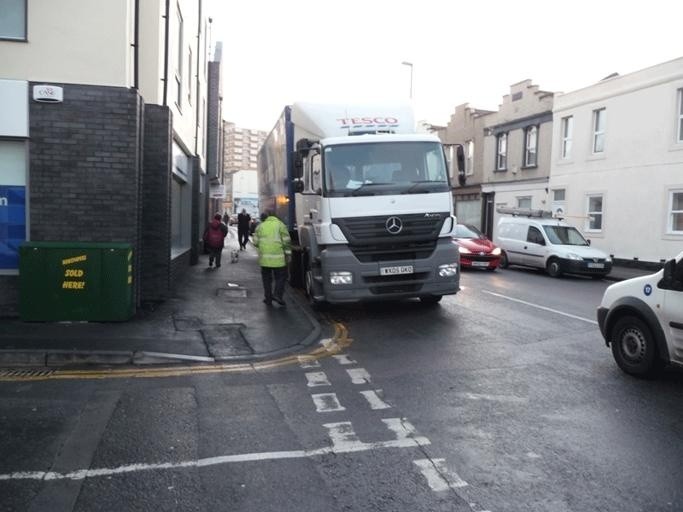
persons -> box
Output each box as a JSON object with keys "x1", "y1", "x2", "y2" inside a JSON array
[
  {"x1": 252, "y1": 207, "x2": 292, "y2": 307},
  {"x1": 236, "y1": 208, "x2": 250, "y2": 251},
  {"x1": 202, "y1": 213, "x2": 227, "y2": 268},
  {"x1": 222, "y1": 212, "x2": 229, "y2": 228}
]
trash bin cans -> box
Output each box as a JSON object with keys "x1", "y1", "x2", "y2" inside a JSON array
[{"x1": 17, "y1": 240, "x2": 135, "y2": 322}]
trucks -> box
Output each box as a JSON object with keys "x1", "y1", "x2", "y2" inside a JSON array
[{"x1": 255, "y1": 101, "x2": 466, "y2": 309}]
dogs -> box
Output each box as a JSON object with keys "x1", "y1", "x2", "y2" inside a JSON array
[{"x1": 230, "y1": 250, "x2": 238, "y2": 263}]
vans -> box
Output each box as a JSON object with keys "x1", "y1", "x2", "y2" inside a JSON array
[{"x1": 494, "y1": 216, "x2": 613, "y2": 280}]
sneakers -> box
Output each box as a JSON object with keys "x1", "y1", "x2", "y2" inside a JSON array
[
  {"x1": 208, "y1": 257, "x2": 221, "y2": 267},
  {"x1": 262, "y1": 296, "x2": 287, "y2": 306}
]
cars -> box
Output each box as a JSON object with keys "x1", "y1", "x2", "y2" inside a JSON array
[
  {"x1": 452, "y1": 222, "x2": 501, "y2": 274},
  {"x1": 597, "y1": 251, "x2": 683, "y2": 376}
]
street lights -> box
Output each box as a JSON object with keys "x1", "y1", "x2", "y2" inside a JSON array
[{"x1": 401, "y1": 61, "x2": 413, "y2": 99}]
